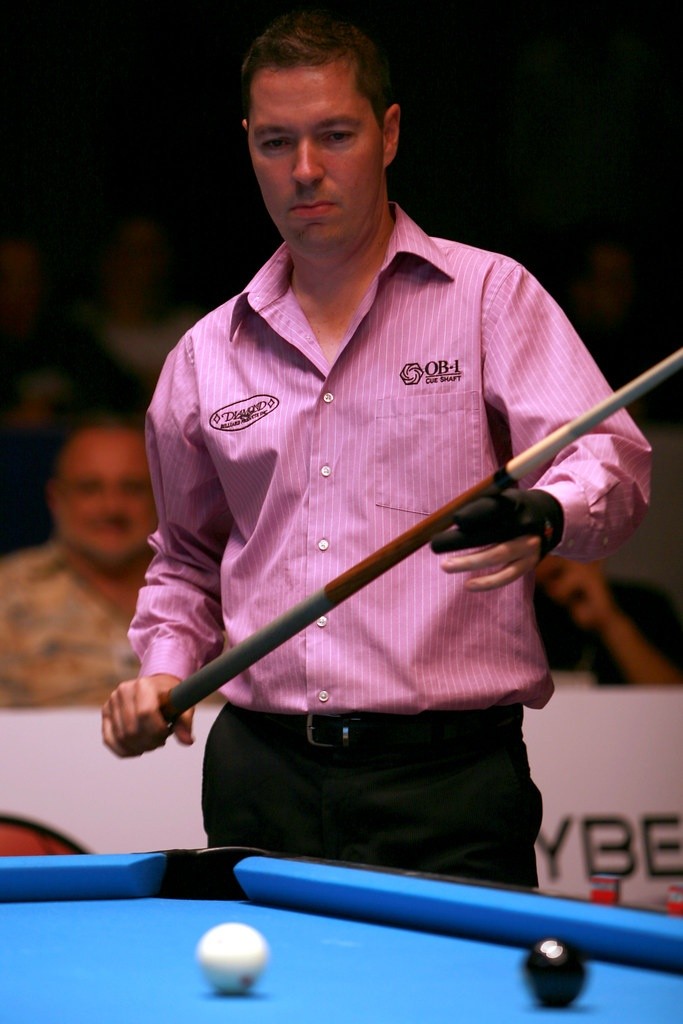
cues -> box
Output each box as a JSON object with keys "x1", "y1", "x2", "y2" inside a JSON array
[{"x1": 159, "y1": 345, "x2": 683, "y2": 724}]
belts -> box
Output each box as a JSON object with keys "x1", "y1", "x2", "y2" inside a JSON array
[{"x1": 251, "y1": 705, "x2": 517, "y2": 747}]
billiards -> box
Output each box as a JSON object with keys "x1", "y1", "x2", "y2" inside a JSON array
[
  {"x1": 522, "y1": 936, "x2": 589, "y2": 1008},
  {"x1": 195, "y1": 922, "x2": 270, "y2": 993}
]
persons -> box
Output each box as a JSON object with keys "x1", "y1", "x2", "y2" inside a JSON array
[
  {"x1": 0, "y1": 416, "x2": 225, "y2": 710},
  {"x1": 0, "y1": 212, "x2": 204, "y2": 555},
  {"x1": 535, "y1": 558, "x2": 682, "y2": 684},
  {"x1": 103, "y1": 9, "x2": 651, "y2": 888}
]
